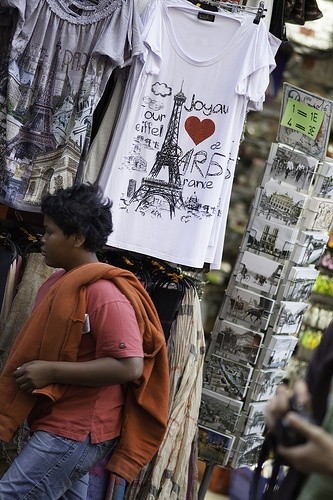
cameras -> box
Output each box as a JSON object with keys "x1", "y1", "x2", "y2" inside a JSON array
[{"x1": 275, "y1": 394, "x2": 316, "y2": 448}]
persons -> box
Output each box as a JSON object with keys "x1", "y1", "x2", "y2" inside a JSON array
[
  {"x1": 264, "y1": 319, "x2": 333, "y2": 500},
  {"x1": 0, "y1": 182, "x2": 144, "y2": 500}
]
